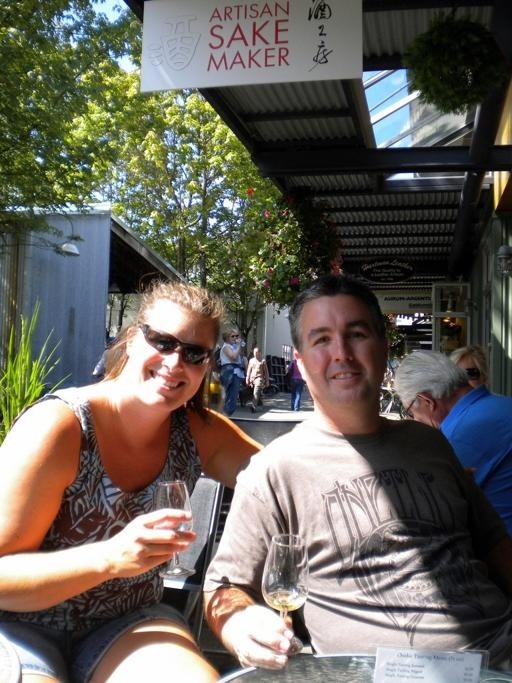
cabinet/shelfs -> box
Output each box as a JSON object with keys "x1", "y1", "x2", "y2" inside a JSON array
[
  {"x1": 426, "y1": 277, "x2": 477, "y2": 355},
  {"x1": 266, "y1": 352, "x2": 289, "y2": 394}
]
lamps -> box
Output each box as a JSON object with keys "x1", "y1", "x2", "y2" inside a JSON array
[{"x1": 493, "y1": 234, "x2": 512, "y2": 282}]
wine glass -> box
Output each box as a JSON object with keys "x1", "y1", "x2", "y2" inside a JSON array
[
  {"x1": 263, "y1": 533, "x2": 310, "y2": 658},
  {"x1": 152, "y1": 480, "x2": 194, "y2": 579}
]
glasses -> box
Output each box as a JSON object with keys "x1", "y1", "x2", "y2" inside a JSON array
[
  {"x1": 139, "y1": 322, "x2": 212, "y2": 363},
  {"x1": 399, "y1": 398, "x2": 418, "y2": 421},
  {"x1": 465, "y1": 367, "x2": 482, "y2": 381}
]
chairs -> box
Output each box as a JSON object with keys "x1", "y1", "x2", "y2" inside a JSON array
[{"x1": 186, "y1": 406, "x2": 316, "y2": 675}]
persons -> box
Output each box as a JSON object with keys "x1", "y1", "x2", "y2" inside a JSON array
[
  {"x1": 220, "y1": 328, "x2": 247, "y2": 418},
  {"x1": 450, "y1": 344, "x2": 488, "y2": 391},
  {"x1": 287, "y1": 357, "x2": 305, "y2": 410},
  {"x1": 392, "y1": 348, "x2": 512, "y2": 540},
  {"x1": 1, "y1": 279, "x2": 266, "y2": 683},
  {"x1": 203, "y1": 274, "x2": 511, "y2": 679},
  {"x1": 246, "y1": 347, "x2": 270, "y2": 413}
]
